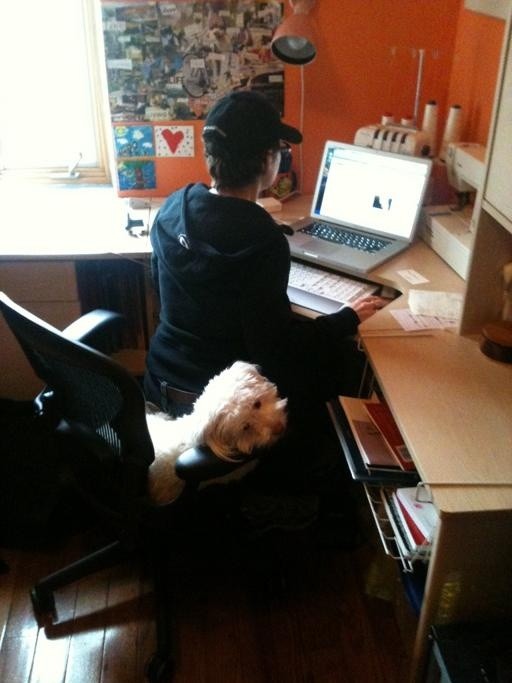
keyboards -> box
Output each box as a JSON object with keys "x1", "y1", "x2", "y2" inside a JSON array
[{"x1": 286, "y1": 259, "x2": 380, "y2": 316}]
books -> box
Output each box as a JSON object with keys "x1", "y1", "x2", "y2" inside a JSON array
[{"x1": 327, "y1": 390, "x2": 438, "y2": 561}]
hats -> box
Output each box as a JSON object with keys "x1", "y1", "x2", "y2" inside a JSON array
[{"x1": 200, "y1": 90, "x2": 304, "y2": 145}]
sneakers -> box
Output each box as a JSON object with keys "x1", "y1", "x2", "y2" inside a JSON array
[{"x1": 235, "y1": 494, "x2": 320, "y2": 539}]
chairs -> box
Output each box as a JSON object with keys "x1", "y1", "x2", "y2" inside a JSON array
[{"x1": 0, "y1": 280, "x2": 329, "y2": 683}]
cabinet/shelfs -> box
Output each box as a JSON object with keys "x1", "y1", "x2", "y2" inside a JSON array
[{"x1": 417, "y1": 0, "x2": 512, "y2": 338}]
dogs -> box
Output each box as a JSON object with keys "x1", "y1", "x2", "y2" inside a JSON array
[{"x1": 96, "y1": 359, "x2": 290, "y2": 512}]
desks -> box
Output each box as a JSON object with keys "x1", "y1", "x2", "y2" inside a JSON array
[{"x1": 0, "y1": 197, "x2": 511, "y2": 682}]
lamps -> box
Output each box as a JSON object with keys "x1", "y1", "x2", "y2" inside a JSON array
[{"x1": 270, "y1": 2, "x2": 315, "y2": 207}]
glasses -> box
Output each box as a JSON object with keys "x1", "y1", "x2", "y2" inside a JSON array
[{"x1": 273, "y1": 142, "x2": 292, "y2": 159}]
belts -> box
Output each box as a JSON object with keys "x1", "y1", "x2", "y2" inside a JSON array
[{"x1": 148, "y1": 372, "x2": 198, "y2": 406}]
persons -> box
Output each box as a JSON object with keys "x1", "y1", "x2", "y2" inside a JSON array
[{"x1": 135, "y1": 90, "x2": 387, "y2": 548}]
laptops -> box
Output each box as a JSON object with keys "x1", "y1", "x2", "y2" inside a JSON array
[{"x1": 281, "y1": 138, "x2": 433, "y2": 275}]
